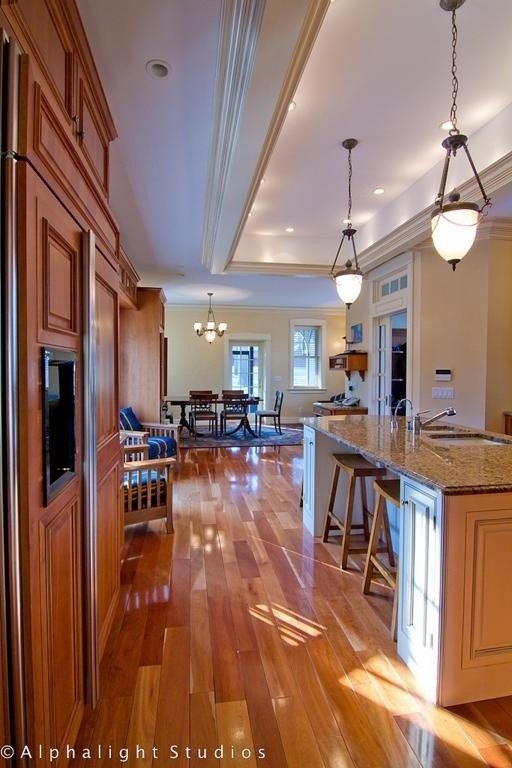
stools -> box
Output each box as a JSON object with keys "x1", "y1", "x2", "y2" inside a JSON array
[
  {"x1": 322, "y1": 453, "x2": 395, "y2": 570},
  {"x1": 360, "y1": 479, "x2": 400, "y2": 642}
]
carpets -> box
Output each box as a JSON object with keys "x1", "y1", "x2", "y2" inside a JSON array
[{"x1": 163, "y1": 422, "x2": 303, "y2": 448}]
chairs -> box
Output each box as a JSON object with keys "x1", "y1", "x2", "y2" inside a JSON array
[
  {"x1": 123, "y1": 444, "x2": 178, "y2": 534},
  {"x1": 255, "y1": 390, "x2": 283, "y2": 436},
  {"x1": 189, "y1": 389, "x2": 249, "y2": 440},
  {"x1": 119, "y1": 406, "x2": 182, "y2": 460}
]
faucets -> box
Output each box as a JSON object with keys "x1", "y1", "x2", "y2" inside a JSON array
[
  {"x1": 393, "y1": 396, "x2": 413, "y2": 437},
  {"x1": 413, "y1": 408, "x2": 458, "y2": 432}
]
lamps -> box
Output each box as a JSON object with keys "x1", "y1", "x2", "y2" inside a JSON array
[
  {"x1": 193, "y1": 292, "x2": 228, "y2": 344},
  {"x1": 430, "y1": 0, "x2": 491, "y2": 273},
  {"x1": 329, "y1": 137, "x2": 363, "y2": 310}
]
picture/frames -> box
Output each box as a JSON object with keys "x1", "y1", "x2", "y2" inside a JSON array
[{"x1": 350, "y1": 322, "x2": 363, "y2": 345}]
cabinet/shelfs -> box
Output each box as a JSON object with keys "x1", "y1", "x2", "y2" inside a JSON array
[
  {"x1": 1, "y1": 0, "x2": 126, "y2": 768},
  {"x1": 120, "y1": 244, "x2": 141, "y2": 311},
  {"x1": 329, "y1": 352, "x2": 368, "y2": 382},
  {"x1": 391, "y1": 328, "x2": 407, "y2": 416},
  {"x1": 120, "y1": 285, "x2": 169, "y2": 424}
]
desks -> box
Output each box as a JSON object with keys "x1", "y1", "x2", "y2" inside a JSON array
[
  {"x1": 163, "y1": 395, "x2": 260, "y2": 438},
  {"x1": 312, "y1": 402, "x2": 368, "y2": 417}
]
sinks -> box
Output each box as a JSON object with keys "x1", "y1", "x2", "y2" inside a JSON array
[
  {"x1": 429, "y1": 433, "x2": 510, "y2": 450},
  {"x1": 401, "y1": 425, "x2": 472, "y2": 432}
]
